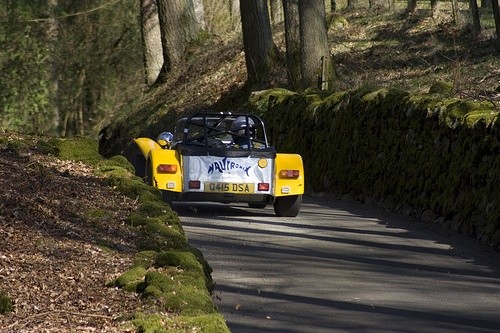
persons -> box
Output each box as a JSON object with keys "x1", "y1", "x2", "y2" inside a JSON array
[{"x1": 226, "y1": 116, "x2": 265, "y2": 150}]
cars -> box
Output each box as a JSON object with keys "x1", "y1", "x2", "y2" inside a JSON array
[{"x1": 129, "y1": 107, "x2": 304, "y2": 218}]
